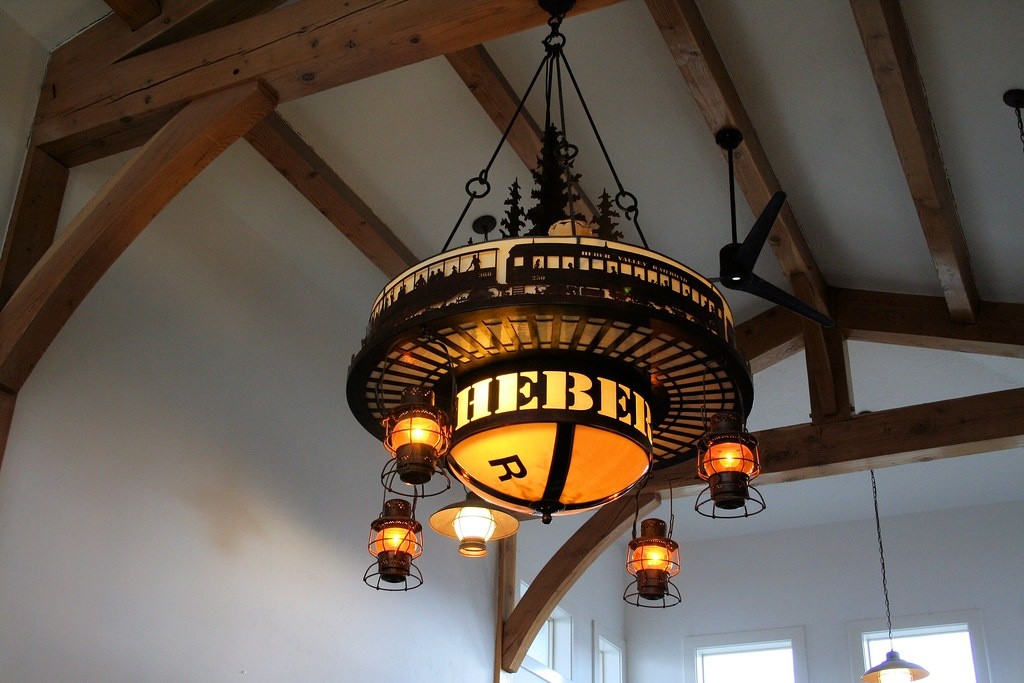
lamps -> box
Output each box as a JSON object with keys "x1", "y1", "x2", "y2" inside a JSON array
[
  {"x1": 622, "y1": 474, "x2": 682, "y2": 609},
  {"x1": 859, "y1": 470, "x2": 929, "y2": 683},
  {"x1": 694, "y1": 362, "x2": 768, "y2": 520},
  {"x1": 363, "y1": 485, "x2": 424, "y2": 591},
  {"x1": 429, "y1": 483, "x2": 520, "y2": 558},
  {"x1": 344, "y1": 0, "x2": 768, "y2": 525},
  {"x1": 380, "y1": 383, "x2": 451, "y2": 498}
]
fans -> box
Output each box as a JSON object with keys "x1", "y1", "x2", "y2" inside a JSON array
[{"x1": 706, "y1": 124, "x2": 834, "y2": 327}]
